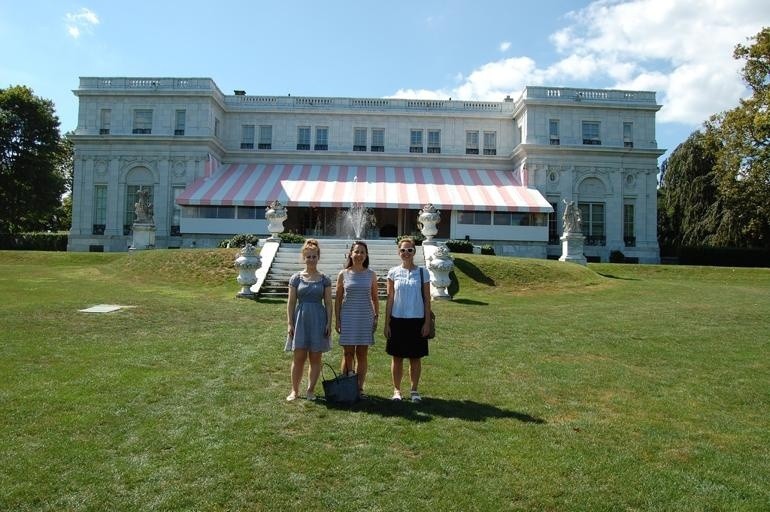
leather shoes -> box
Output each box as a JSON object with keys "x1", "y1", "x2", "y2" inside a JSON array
[
  {"x1": 286, "y1": 395, "x2": 298, "y2": 401},
  {"x1": 306, "y1": 391, "x2": 317, "y2": 400}
]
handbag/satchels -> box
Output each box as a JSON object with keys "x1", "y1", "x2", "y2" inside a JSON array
[
  {"x1": 321, "y1": 351, "x2": 360, "y2": 406},
  {"x1": 428, "y1": 312, "x2": 436, "y2": 339}
]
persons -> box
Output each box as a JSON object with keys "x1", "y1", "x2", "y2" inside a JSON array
[
  {"x1": 333, "y1": 239, "x2": 381, "y2": 402},
  {"x1": 383, "y1": 236, "x2": 432, "y2": 402},
  {"x1": 281, "y1": 237, "x2": 335, "y2": 402}
]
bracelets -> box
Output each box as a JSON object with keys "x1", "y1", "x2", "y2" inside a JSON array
[{"x1": 374, "y1": 312, "x2": 379, "y2": 318}]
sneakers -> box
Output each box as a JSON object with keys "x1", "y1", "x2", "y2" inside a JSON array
[
  {"x1": 410, "y1": 391, "x2": 422, "y2": 403},
  {"x1": 393, "y1": 390, "x2": 402, "y2": 401}
]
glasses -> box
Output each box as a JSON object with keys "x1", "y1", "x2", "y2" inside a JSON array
[{"x1": 400, "y1": 248, "x2": 416, "y2": 253}]
